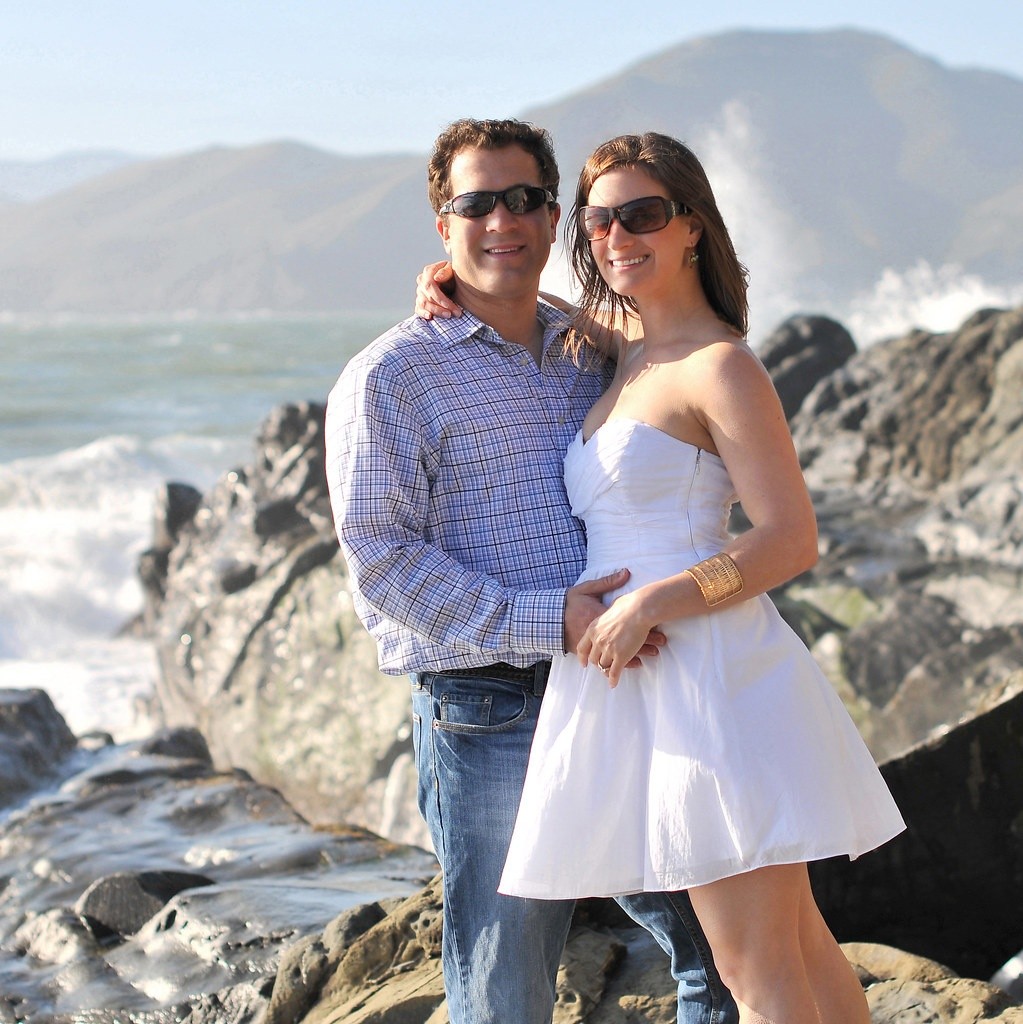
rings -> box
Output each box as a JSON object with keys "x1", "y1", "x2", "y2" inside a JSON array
[{"x1": 598, "y1": 662, "x2": 606, "y2": 672}]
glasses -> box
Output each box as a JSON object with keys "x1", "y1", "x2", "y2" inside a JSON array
[
  {"x1": 438, "y1": 186, "x2": 556, "y2": 219},
  {"x1": 577, "y1": 195, "x2": 692, "y2": 241}
]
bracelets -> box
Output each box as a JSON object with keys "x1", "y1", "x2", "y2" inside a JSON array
[{"x1": 684, "y1": 551, "x2": 744, "y2": 606}]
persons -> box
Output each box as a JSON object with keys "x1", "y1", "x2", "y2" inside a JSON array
[
  {"x1": 325, "y1": 118, "x2": 741, "y2": 1024},
  {"x1": 414, "y1": 133, "x2": 907, "y2": 1024}
]
